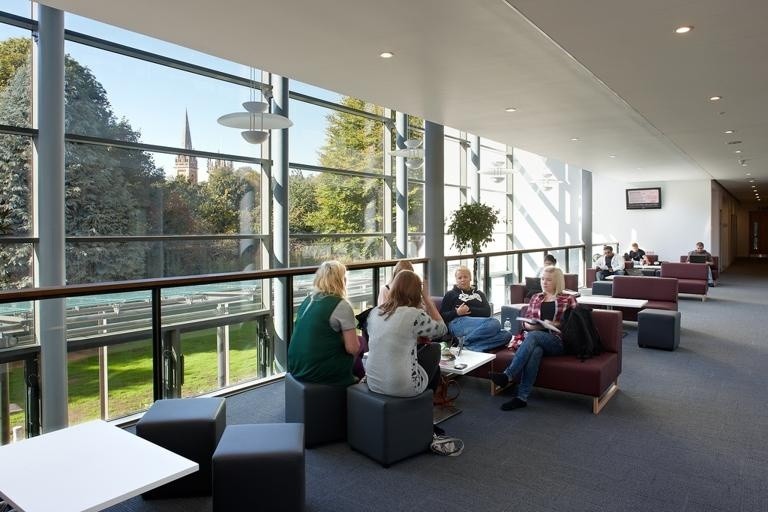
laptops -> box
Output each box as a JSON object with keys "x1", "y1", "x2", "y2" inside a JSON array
[{"x1": 690, "y1": 255, "x2": 706, "y2": 263}]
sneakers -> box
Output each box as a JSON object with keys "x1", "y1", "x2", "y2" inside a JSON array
[
  {"x1": 501, "y1": 398, "x2": 527, "y2": 410},
  {"x1": 488, "y1": 371, "x2": 509, "y2": 388},
  {"x1": 429, "y1": 432, "x2": 464, "y2": 457},
  {"x1": 451, "y1": 335, "x2": 462, "y2": 347}
]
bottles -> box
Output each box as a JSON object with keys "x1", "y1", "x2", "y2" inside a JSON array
[{"x1": 503, "y1": 318, "x2": 512, "y2": 332}]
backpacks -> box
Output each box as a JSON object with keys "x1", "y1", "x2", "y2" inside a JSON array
[{"x1": 560, "y1": 295, "x2": 601, "y2": 360}]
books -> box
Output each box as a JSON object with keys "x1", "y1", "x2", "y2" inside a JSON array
[{"x1": 515, "y1": 315, "x2": 561, "y2": 334}]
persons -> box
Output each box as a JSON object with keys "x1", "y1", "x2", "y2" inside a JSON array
[
  {"x1": 537, "y1": 254, "x2": 556, "y2": 276},
  {"x1": 363, "y1": 269, "x2": 465, "y2": 437},
  {"x1": 378, "y1": 259, "x2": 426, "y2": 311},
  {"x1": 488, "y1": 267, "x2": 576, "y2": 411},
  {"x1": 439, "y1": 266, "x2": 512, "y2": 352},
  {"x1": 630, "y1": 243, "x2": 651, "y2": 266},
  {"x1": 593, "y1": 247, "x2": 625, "y2": 282},
  {"x1": 287, "y1": 261, "x2": 368, "y2": 386},
  {"x1": 686, "y1": 243, "x2": 714, "y2": 287}
]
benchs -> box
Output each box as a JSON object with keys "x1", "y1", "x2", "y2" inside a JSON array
[
  {"x1": 490, "y1": 306, "x2": 623, "y2": 415},
  {"x1": 424, "y1": 295, "x2": 493, "y2": 395},
  {"x1": 500, "y1": 250, "x2": 721, "y2": 332}
]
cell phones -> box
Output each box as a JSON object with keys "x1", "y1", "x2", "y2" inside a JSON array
[{"x1": 455, "y1": 364, "x2": 467, "y2": 369}]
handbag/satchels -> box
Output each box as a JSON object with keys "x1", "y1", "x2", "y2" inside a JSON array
[
  {"x1": 508, "y1": 328, "x2": 529, "y2": 352},
  {"x1": 432, "y1": 374, "x2": 461, "y2": 409},
  {"x1": 625, "y1": 267, "x2": 644, "y2": 276}
]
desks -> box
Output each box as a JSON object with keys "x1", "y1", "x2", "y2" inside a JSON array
[
  {"x1": 431, "y1": 343, "x2": 499, "y2": 426},
  {"x1": 0, "y1": 417, "x2": 204, "y2": 512}
]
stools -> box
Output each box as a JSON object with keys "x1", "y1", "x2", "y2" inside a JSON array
[
  {"x1": 348, "y1": 380, "x2": 434, "y2": 467},
  {"x1": 136, "y1": 398, "x2": 225, "y2": 501},
  {"x1": 213, "y1": 423, "x2": 305, "y2": 509},
  {"x1": 635, "y1": 307, "x2": 683, "y2": 351},
  {"x1": 283, "y1": 370, "x2": 348, "y2": 447}
]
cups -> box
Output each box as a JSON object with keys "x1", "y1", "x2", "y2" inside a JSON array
[{"x1": 439, "y1": 335, "x2": 468, "y2": 368}]
